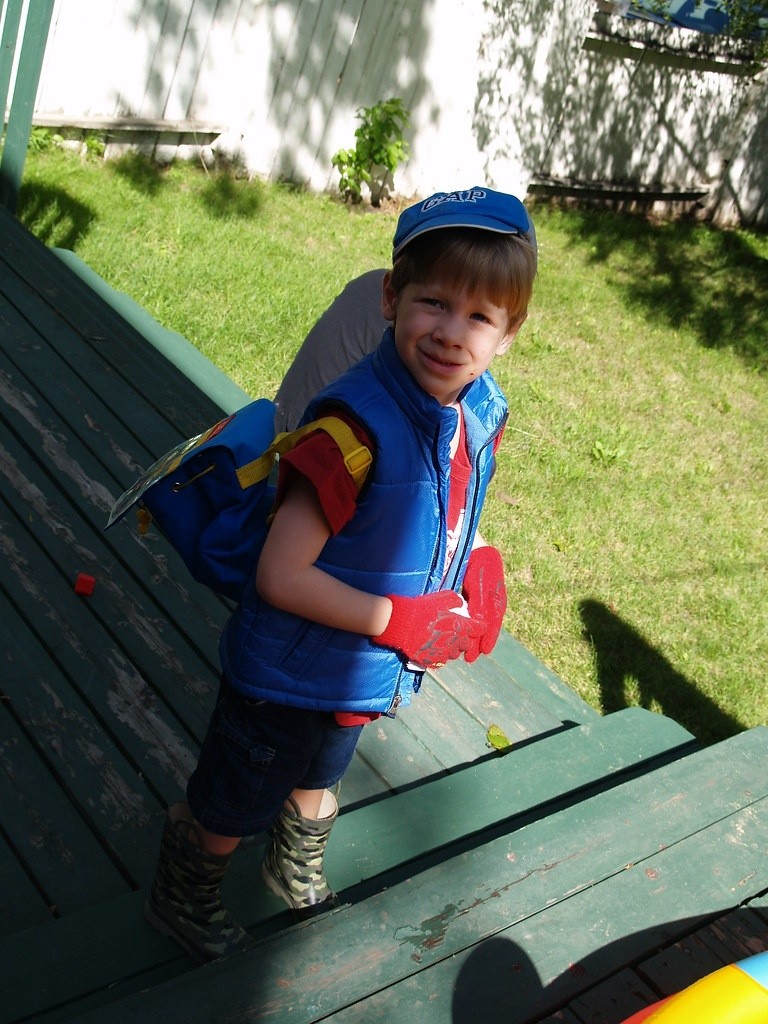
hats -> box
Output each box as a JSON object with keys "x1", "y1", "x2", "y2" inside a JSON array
[{"x1": 393, "y1": 187, "x2": 528, "y2": 262}]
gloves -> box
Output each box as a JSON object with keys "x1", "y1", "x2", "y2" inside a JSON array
[
  {"x1": 372, "y1": 589, "x2": 489, "y2": 668},
  {"x1": 461, "y1": 546, "x2": 505, "y2": 661}
]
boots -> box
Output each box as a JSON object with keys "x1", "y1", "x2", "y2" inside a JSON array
[
  {"x1": 260, "y1": 805, "x2": 343, "y2": 925},
  {"x1": 146, "y1": 801, "x2": 259, "y2": 969}
]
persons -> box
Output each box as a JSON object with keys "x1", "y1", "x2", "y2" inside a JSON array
[
  {"x1": 142, "y1": 186, "x2": 538, "y2": 965},
  {"x1": 273, "y1": 268, "x2": 392, "y2": 434}
]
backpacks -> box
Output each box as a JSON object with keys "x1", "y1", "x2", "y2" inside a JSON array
[{"x1": 103, "y1": 400, "x2": 374, "y2": 610}]
